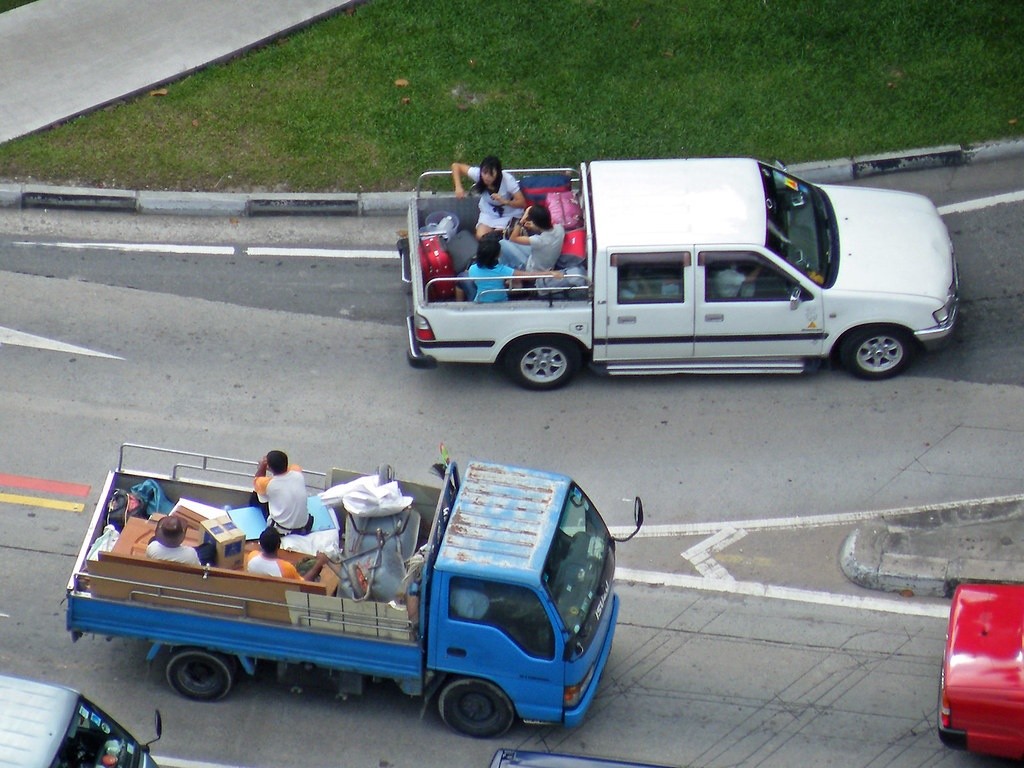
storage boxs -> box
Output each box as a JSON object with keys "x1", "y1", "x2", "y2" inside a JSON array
[{"x1": 198, "y1": 515, "x2": 247, "y2": 569}]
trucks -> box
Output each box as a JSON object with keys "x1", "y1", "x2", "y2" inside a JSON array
[{"x1": 60, "y1": 440, "x2": 643, "y2": 744}]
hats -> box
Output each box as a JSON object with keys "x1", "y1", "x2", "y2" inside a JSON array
[{"x1": 155, "y1": 516, "x2": 188, "y2": 547}]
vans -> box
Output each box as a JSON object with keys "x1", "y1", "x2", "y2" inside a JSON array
[{"x1": 1, "y1": 678, "x2": 161, "y2": 768}]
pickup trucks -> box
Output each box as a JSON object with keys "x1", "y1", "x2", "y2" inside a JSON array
[{"x1": 395, "y1": 159, "x2": 962, "y2": 391}]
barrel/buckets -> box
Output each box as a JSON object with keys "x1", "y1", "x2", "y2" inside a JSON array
[
  {"x1": 557, "y1": 226, "x2": 586, "y2": 268},
  {"x1": 425, "y1": 211, "x2": 459, "y2": 240}
]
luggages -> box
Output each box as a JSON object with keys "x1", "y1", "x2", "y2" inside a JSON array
[
  {"x1": 418, "y1": 234, "x2": 455, "y2": 298},
  {"x1": 546, "y1": 191, "x2": 584, "y2": 230}
]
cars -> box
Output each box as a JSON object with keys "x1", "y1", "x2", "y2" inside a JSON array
[{"x1": 937, "y1": 580, "x2": 1024, "y2": 760}]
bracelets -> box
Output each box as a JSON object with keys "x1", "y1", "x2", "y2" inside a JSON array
[{"x1": 517, "y1": 218, "x2": 525, "y2": 227}]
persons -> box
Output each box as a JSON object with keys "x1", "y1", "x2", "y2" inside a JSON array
[
  {"x1": 469, "y1": 235, "x2": 566, "y2": 304},
  {"x1": 249, "y1": 450, "x2": 314, "y2": 536},
  {"x1": 499, "y1": 204, "x2": 565, "y2": 272},
  {"x1": 710, "y1": 262, "x2": 762, "y2": 298},
  {"x1": 246, "y1": 526, "x2": 329, "y2": 582},
  {"x1": 452, "y1": 155, "x2": 528, "y2": 240},
  {"x1": 145, "y1": 516, "x2": 217, "y2": 568},
  {"x1": 619, "y1": 267, "x2": 653, "y2": 299}
]
